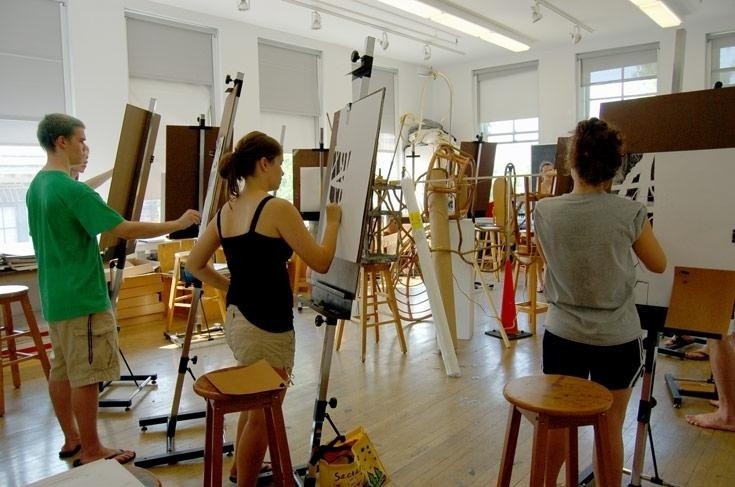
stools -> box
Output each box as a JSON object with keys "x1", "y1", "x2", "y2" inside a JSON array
[
  {"x1": 193, "y1": 366, "x2": 298, "y2": 485},
  {"x1": 163, "y1": 247, "x2": 228, "y2": 343},
  {"x1": 336, "y1": 256, "x2": 409, "y2": 363},
  {"x1": 475, "y1": 225, "x2": 503, "y2": 282},
  {"x1": 495, "y1": 373, "x2": 619, "y2": 487},
  {"x1": 509, "y1": 255, "x2": 552, "y2": 336},
  {"x1": 0, "y1": 280, "x2": 55, "y2": 418}
]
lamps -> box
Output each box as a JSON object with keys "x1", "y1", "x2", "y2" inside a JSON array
[
  {"x1": 528, "y1": 3, "x2": 543, "y2": 26},
  {"x1": 422, "y1": 44, "x2": 435, "y2": 63},
  {"x1": 237, "y1": 0, "x2": 250, "y2": 14},
  {"x1": 311, "y1": 11, "x2": 326, "y2": 32},
  {"x1": 568, "y1": 23, "x2": 584, "y2": 45},
  {"x1": 377, "y1": 30, "x2": 393, "y2": 51}
]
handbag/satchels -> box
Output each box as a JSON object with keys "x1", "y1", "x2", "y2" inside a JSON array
[{"x1": 308, "y1": 425, "x2": 391, "y2": 486}]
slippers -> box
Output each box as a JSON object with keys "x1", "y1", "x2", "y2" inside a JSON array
[
  {"x1": 229, "y1": 461, "x2": 274, "y2": 483},
  {"x1": 72, "y1": 449, "x2": 136, "y2": 468},
  {"x1": 683, "y1": 350, "x2": 710, "y2": 362},
  {"x1": 664, "y1": 335, "x2": 696, "y2": 350},
  {"x1": 58, "y1": 444, "x2": 82, "y2": 459}
]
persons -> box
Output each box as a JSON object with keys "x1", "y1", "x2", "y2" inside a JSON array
[
  {"x1": 685, "y1": 328, "x2": 735, "y2": 433},
  {"x1": 22, "y1": 114, "x2": 203, "y2": 470},
  {"x1": 534, "y1": 117, "x2": 667, "y2": 487},
  {"x1": 68, "y1": 145, "x2": 116, "y2": 188},
  {"x1": 661, "y1": 333, "x2": 708, "y2": 361},
  {"x1": 536, "y1": 162, "x2": 555, "y2": 293},
  {"x1": 186, "y1": 130, "x2": 342, "y2": 486}
]
